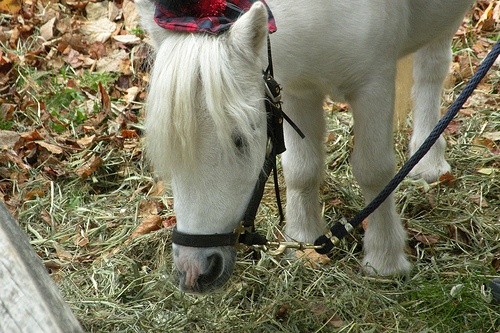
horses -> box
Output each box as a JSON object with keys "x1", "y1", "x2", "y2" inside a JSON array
[{"x1": 133, "y1": 0, "x2": 475, "y2": 296}]
